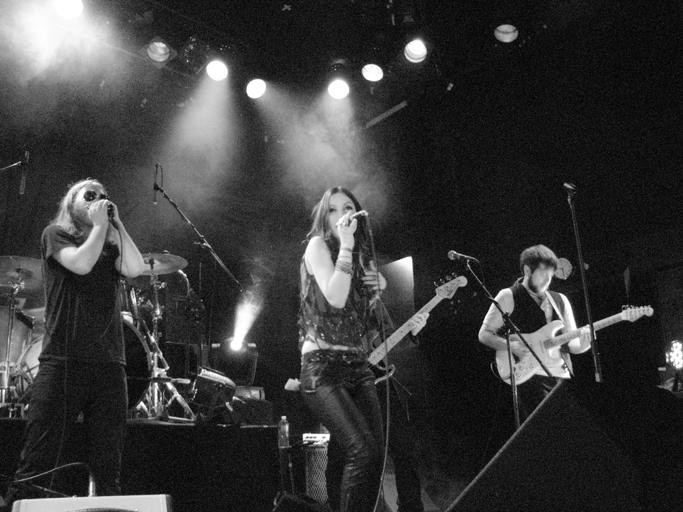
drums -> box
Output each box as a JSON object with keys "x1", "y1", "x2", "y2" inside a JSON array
[
  {"x1": 0, "y1": 306, "x2": 44, "y2": 397},
  {"x1": 123, "y1": 319, "x2": 152, "y2": 409}
]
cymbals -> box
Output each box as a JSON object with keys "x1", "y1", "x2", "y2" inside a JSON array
[
  {"x1": 1, "y1": 255, "x2": 43, "y2": 291},
  {"x1": 140, "y1": 252, "x2": 188, "y2": 276}
]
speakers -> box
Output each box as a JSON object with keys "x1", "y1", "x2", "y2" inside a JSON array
[
  {"x1": 120, "y1": 423, "x2": 284, "y2": 512},
  {"x1": 444, "y1": 380, "x2": 683, "y2": 512}
]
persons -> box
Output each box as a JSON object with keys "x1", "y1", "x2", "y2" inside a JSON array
[
  {"x1": 3, "y1": 180, "x2": 147, "y2": 512},
  {"x1": 476, "y1": 244, "x2": 598, "y2": 416},
  {"x1": 295, "y1": 184, "x2": 391, "y2": 511},
  {"x1": 356, "y1": 291, "x2": 428, "y2": 511}
]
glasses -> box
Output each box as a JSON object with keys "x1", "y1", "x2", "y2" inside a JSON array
[{"x1": 78, "y1": 189, "x2": 111, "y2": 204}]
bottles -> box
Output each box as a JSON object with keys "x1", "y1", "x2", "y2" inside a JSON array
[{"x1": 279, "y1": 414, "x2": 290, "y2": 447}]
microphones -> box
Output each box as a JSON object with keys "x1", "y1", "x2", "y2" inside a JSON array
[
  {"x1": 153, "y1": 164, "x2": 159, "y2": 205},
  {"x1": 19, "y1": 151, "x2": 30, "y2": 195},
  {"x1": 107, "y1": 205, "x2": 123, "y2": 235},
  {"x1": 448, "y1": 250, "x2": 479, "y2": 265},
  {"x1": 349, "y1": 209, "x2": 368, "y2": 221}
]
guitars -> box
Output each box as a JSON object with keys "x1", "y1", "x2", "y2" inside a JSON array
[
  {"x1": 366, "y1": 273, "x2": 468, "y2": 385},
  {"x1": 495, "y1": 305, "x2": 654, "y2": 386}
]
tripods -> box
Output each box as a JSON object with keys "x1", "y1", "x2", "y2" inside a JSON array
[{"x1": 135, "y1": 313, "x2": 197, "y2": 421}]
